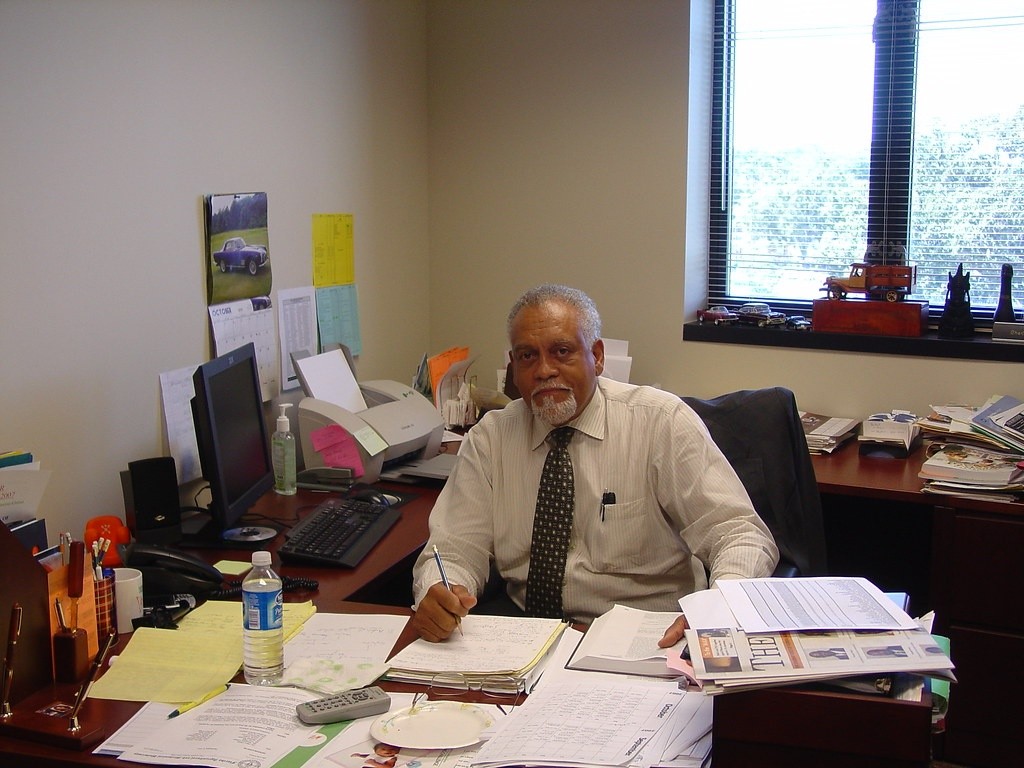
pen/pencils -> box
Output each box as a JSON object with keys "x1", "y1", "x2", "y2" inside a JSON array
[
  {"x1": 1, "y1": 602, "x2": 23, "y2": 703},
  {"x1": 66, "y1": 532, "x2": 72, "y2": 545},
  {"x1": 60, "y1": 533, "x2": 65, "y2": 566},
  {"x1": 54, "y1": 598, "x2": 67, "y2": 633},
  {"x1": 166, "y1": 683, "x2": 231, "y2": 720},
  {"x1": 432, "y1": 544, "x2": 464, "y2": 637},
  {"x1": 70, "y1": 627, "x2": 116, "y2": 717},
  {"x1": 92, "y1": 537, "x2": 111, "y2": 581}
]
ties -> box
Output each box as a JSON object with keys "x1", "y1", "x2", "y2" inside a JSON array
[{"x1": 524, "y1": 425, "x2": 576, "y2": 619}]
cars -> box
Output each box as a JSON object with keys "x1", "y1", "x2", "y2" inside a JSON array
[
  {"x1": 213, "y1": 236, "x2": 270, "y2": 276},
  {"x1": 696, "y1": 306, "x2": 739, "y2": 327},
  {"x1": 784, "y1": 315, "x2": 812, "y2": 331}
]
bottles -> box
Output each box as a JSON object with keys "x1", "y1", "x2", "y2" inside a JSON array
[
  {"x1": 992, "y1": 263, "x2": 1016, "y2": 331},
  {"x1": 241, "y1": 551, "x2": 285, "y2": 686}
]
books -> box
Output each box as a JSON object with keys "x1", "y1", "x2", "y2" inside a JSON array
[
  {"x1": 565, "y1": 603, "x2": 702, "y2": 685},
  {"x1": 799, "y1": 410, "x2": 862, "y2": 456},
  {"x1": 916, "y1": 393, "x2": 1024, "y2": 505}
]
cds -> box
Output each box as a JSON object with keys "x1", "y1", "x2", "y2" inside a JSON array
[{"x1": 223, "y1": 527, "x2": 277, "y2": 541}]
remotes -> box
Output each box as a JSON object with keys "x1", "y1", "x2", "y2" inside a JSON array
[{"x1": 295, "y1": 687, "x2": 391, "y2": 724}]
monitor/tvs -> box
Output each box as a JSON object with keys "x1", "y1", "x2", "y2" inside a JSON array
[{"x1": 177, "y1": 341, "x2": 285, "y2": 551}]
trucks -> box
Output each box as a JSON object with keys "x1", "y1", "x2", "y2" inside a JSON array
[{"x1": 817, "y1": 261, "x2": 917, "y2": 302}]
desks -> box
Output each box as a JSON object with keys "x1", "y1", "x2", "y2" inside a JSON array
[{"x1": 0, "y1": 418, "x2": 1022, "y2": 768}]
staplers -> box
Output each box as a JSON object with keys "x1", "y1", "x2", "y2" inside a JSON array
[{"x1": 296, "y1": 467, "x2": 355, "y2": 493}]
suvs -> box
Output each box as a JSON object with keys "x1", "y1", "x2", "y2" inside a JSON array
[{"x1": 728, "y1": 302, "x2": 786, "y2": 329}]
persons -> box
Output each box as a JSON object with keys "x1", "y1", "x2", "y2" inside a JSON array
[
  {"x1": 351, "y1": 743, "x2": 401, "y2": 768},
  {"x1": 867, "y1": 646, "x2": 908, "y2": 657},
  {"x1": 408, "y1": 286, "x2": 780, "y2": 667},
  {"x1": 925, "y1": 647, "x2": 944, "y2": 653},
  {"x1": 808, "y1": 648, "x2": 850, "y2": 660},
  {"x1": 800, "y1": 630, "x2": 832, "y2": 637},
  {"x1": 855, "y1": 630, "x2": 894, "y2": 635}
]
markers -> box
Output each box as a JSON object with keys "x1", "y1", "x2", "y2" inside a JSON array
[{"x1": 104, "y1": 568, "x2": 113, "y2": 578}]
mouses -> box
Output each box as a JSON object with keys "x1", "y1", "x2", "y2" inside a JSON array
[{"x1": 353, "y1": 489, "x2": 391, "y2": 508}]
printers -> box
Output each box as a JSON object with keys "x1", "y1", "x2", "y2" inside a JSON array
[{"x1": 273, "y1": 342, "x2": 461, "y2": 484}]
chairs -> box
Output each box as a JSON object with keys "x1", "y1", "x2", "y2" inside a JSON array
[{"x1": 675, "y1": 384, "x2": 833, "y2": 585}]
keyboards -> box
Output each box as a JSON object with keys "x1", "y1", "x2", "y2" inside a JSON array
[{"x1": 278, "y1": 498, "x2": 401, "y2": 570}]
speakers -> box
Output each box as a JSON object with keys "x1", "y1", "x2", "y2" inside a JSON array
[{"x1": 128, "y1": 457, "x2": 182, "y2": 550}]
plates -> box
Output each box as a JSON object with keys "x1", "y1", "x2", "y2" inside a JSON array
[{"x1": 370, "y1": 700, "x2": 498, "y2": 750}]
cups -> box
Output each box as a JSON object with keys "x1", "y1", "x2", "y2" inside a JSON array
[
  {"x1": 94, "y1": 568, "x2": 122, "y2": 650},
  {"x1": 113, "y1": 568, "x2": 144, "y2": 634}
]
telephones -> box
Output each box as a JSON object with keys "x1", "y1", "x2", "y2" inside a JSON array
[{"x1": 126, "y1": 542, "x2": 225, "y2": 600}]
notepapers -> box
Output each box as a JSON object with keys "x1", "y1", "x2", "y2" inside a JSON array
[{"x1": 213, "y1": 560, "x2": 254, "y2": 576}]
